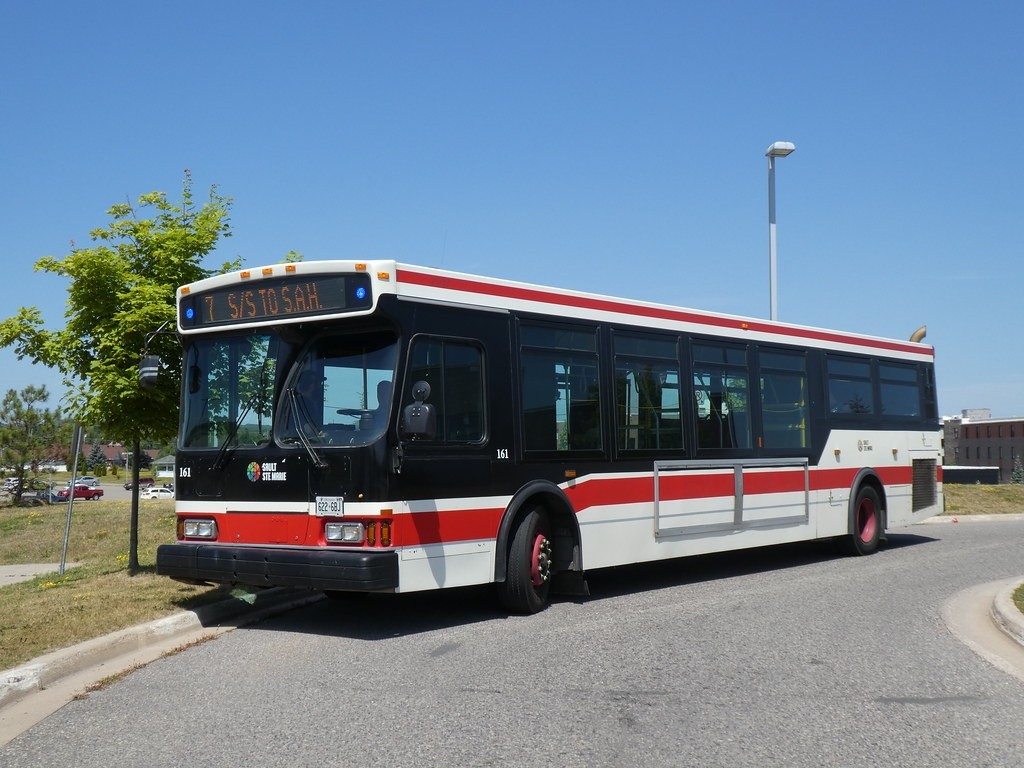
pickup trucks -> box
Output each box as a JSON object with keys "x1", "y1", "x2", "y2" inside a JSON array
[
  {"x1": 57, "y1": 484, "x2": 104, "y2": 501},
  {"x1": 21, "y1": 490, "x2": 67, "y2": 502}
]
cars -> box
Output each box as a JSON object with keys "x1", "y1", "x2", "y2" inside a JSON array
[
  {"x1": 4, "y1": 477, "x2": 50, "y2": 492},
  {"x1": 67, "y1": 476, "x2": 100, "y2": 488}
]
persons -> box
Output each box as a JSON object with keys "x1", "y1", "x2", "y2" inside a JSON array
[
  {"x1": 281, "y1": 369, "x2": 322, "y2": 436},
  {"x1": 374, "y1": 380, "x2": 392, "y2": 430}
]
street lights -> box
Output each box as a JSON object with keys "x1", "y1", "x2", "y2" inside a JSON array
[
  {"x1": 765, "y1": 140, "x2": 796, "y2": 322},
  {"x1": 122, "y1": 452, "x2": 133, "y2": 484}
]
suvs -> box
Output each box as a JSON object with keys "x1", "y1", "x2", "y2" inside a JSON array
[
  {"x1": 124, "y1": 477, "x2": 155, "y2": 491},
  {"x1": 21, "y1": 458, "x2": 57, "y2": 474},
  {"x1": 141, "y1": 487, "x2": 175, "y2": 501}
]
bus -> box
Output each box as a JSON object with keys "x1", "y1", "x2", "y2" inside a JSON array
[{"x1": 139, "y1": 260, "x2": 945, "y2": 617}]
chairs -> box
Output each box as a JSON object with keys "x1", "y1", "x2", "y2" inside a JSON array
[{"x1": 652, "y1": 398, "x2": 874, "y2": 450}]
